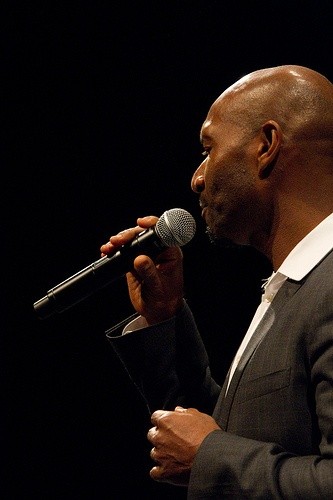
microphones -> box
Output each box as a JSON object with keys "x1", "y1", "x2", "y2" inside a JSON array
[{"x1": 33, "y1": 208, "x2": 196, "y2": 320}]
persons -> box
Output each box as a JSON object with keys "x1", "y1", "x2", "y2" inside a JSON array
[{"x1": 102, "y1": 66, "x2": 333, "y2": 500}]
